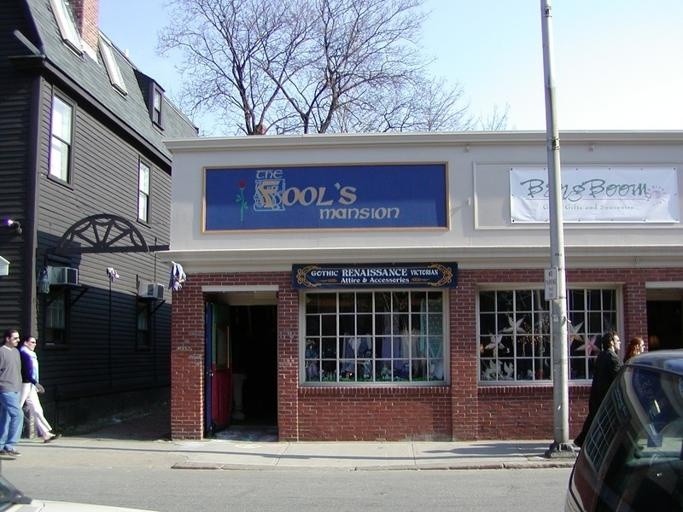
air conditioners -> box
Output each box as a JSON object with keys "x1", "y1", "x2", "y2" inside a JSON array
[
  {"x1": 52, "y1": 267, "x2": 78, "y2": 285},
  {"x1": 144, "y1": 282, "x2": 164, "y2": 300}
]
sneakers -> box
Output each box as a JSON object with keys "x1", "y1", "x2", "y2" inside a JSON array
[
  {"x1": 44, "y1": 433, "x2": 62, "y2": 443},
  {"x1": 0, "y1": 450, "x2": 22, "y2": 460},
  {"x1": 571, "y1": 442, "x2": 581, "y2": 452}
]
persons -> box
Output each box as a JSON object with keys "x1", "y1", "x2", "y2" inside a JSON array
[
  {"x1": 573, "y1": 331, "x2": 646, "y2": 447},
  {"x1": 0, "y1": 329, "x2": 62, "y2": 457},
  {"x1": 400, "y1": 321, "x2": 425, "y2": 378}
]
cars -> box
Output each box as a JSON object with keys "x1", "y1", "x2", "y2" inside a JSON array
[
  {"x1": 0, "y1": 474, "x2": 162, "y2": 512},
  {"x1": 563, "y1": 348, "x2": 683, "y2": 512}
]
